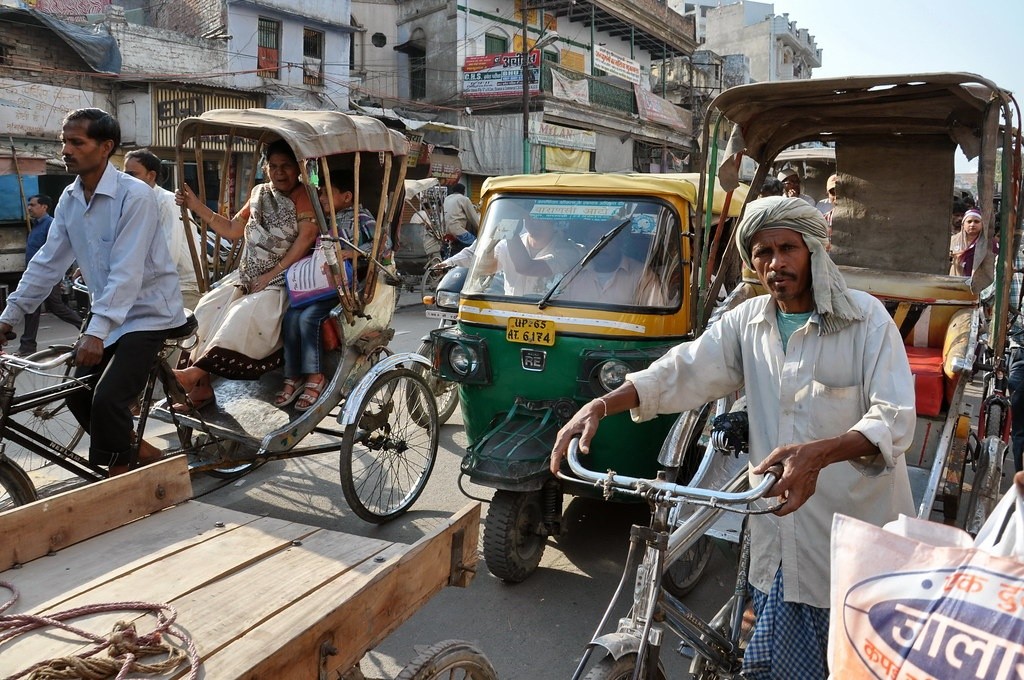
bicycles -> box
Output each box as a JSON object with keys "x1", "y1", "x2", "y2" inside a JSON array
[{"x1": 978, "y1": 339, "x2": 1011, "y2": 456}]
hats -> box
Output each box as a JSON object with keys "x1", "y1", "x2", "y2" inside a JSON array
[
  {"x1": 778, "y1": 169, "x2": 797, "y2": 182},
  {"x1": 963, "y1": 210, "x2": 982, "y2": 221},
  {"x1": 827, "y1": 174, "x2": 836, "y2": 191}
]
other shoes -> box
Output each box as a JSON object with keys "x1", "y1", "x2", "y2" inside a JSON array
[{"x1": 11, "y1": 347, "x2": 37, "y2": 357}]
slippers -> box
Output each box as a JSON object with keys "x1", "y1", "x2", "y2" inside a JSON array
[{"x1": 159, "y1": 361, "x2": 215, "y2": 414}]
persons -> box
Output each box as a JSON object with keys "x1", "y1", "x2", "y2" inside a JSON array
[
  {"x1": 949, "y1": 189, "x2": 1024, "y2": 495},
  {"x1": 123, "y1": 149, "x2": 204, "y2": 413},
  {"x1": 165, "y1": 138, "x2": 321, "y2": 414},
  {"x1": 758, "y1": 169, "x2": 837, "y2": 214},
  {"x1": 411, "y1": 184, "x2": 727, "y2": 315},
  {"x1": 0, "y1": 109, "x2": 188, "y2": 478},
  {"x1": 273, "y1": 168, "x2": 392, "y2": 412},
  {"x1": 550, "y1": 196, "x2": 916, "y2": 680},
  {"x1": 10, "y1": 194, "x2": 86, "y2": 356}
]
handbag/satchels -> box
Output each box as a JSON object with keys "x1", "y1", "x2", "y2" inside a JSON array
[
  {"x1": 284, "y1": 248, "x2": 354, "y2": 309},
  {"x1": 827, "y1": 484, "x2": 1024, "y2": 679}
]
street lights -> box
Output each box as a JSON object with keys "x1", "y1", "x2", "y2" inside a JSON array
[{"x1": 523, "y1": 30, "x2": 560, "y2": 173}]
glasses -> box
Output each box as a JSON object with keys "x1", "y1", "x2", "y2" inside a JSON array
[{"x1": 784, "y1": 180, "x2": 800, "y2": 185}]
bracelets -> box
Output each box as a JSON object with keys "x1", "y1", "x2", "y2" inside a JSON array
[
  {"x1": 592, "y1": 398, "x2": 607, "y2": 420},
  {"x1": 206, "y1": 212, "x2": 217, "y2": 223}
]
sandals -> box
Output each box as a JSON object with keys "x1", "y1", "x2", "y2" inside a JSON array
[
  {"x1": 294, "y1": 374, "x2": 330, "y2": 412},
  {"x1": 273, "y1": 377, "x2": 306, "y2": 406}
]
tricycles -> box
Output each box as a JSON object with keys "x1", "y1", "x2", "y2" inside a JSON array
[
  {"x1": 555, "y1": 73, "x2": 1023, "y2": 679},
  {"x1": 1, "y1": 108, "x2": 443, "y2": 524},
  {"x1": 395, "y1": 177, "x2": 448, "y2": 311}
]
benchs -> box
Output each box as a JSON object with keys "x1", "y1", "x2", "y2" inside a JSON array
[
  {"x1": 745, "y1": 261, "x2": 978, "y2": 330},
  {"x1": 223, "y1": 233, "x2": 395, "y2": 344}
]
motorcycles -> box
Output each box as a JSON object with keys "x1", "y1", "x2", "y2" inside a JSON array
[
  {"x1": 406, "y1": 266, "x2": 468, "y2": 428},
  {"x1": 429, "y1": 174, "x2": 751, "y2": 584}
]
